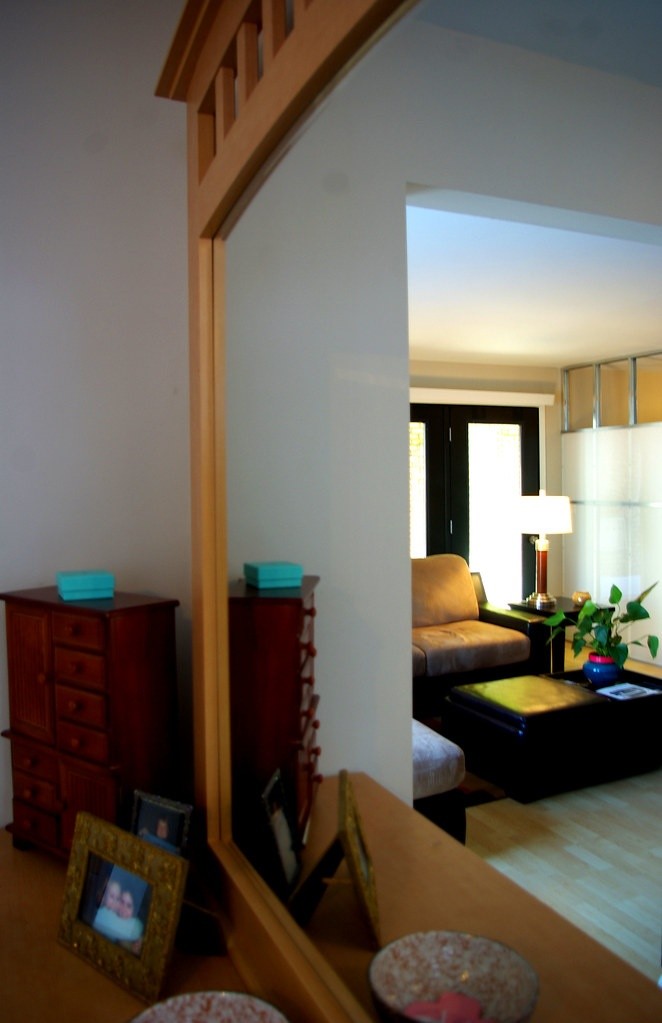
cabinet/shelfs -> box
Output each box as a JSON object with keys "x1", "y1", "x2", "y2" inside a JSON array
[
  {"x1": 0, "y1": 590, "x2": 182, "y2": 866},
  {"x1": 228, "y1": 575, "x2": 323, "y2": 847}
]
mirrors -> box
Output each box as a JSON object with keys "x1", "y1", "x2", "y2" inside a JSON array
[{"x1": 207, "y1": 0, "x2": 662, "y2": 1023}]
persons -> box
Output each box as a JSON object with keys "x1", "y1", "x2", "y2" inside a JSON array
[
  {"x1": 83, "y1": 880, "x2": 143, "y2": 954},
  {"x1": 139, "y1": 818, "x2": 167, "y2": 841}
]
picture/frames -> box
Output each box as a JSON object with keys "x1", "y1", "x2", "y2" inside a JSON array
[
  {"x1": 253, "y1": 766, "x2": 302, "y2": 903},
  {"x1": 56, "y1": 810, "x2": 192, "y2": 1005},
  {"x1": 305, "y1": 767, "x2": 391, "y2": 948},
  {"x1": 128, "y1": 787, "x2": 199, "y2": 852}
]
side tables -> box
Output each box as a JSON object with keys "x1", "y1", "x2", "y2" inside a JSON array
[{"x1": 506, "y1": 597, "x2": 617, "y2": 678}]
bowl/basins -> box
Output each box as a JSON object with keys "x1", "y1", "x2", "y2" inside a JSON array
[
  {"x1": 129, "y1": 990, "x2": 292, "y2": 1023},
  {"x1": 367, "y1": 929, "x2": 539, "y2": 1023}
]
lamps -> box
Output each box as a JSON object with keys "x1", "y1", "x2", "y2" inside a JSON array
[{"x1": 520, "y1": 493, "x2": 574, "y2": 607}]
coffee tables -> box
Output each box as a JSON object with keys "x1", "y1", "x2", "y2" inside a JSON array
[{"x1": 438, "y1": 667, "x2": 662, "y2": 806}]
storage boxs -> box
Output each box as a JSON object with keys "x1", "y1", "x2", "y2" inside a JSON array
[
  {"x1": 54, "y1": 569, "x2": 117, "y2": 601},
  {"x1": 244, "y1": 561, "x2": 305, "y2": 589}
]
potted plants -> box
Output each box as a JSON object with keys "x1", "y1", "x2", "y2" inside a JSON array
[{"x1": 543, "y1": 579, "x2": 660, "y2": 686}]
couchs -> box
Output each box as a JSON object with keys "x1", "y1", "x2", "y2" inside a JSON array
[
  {"x1": 413, "y1": 550, "x2": 548, "y2": 719},
  {"x1": 414, "y1": 718, "x2": 467, "y2": 846}
]
